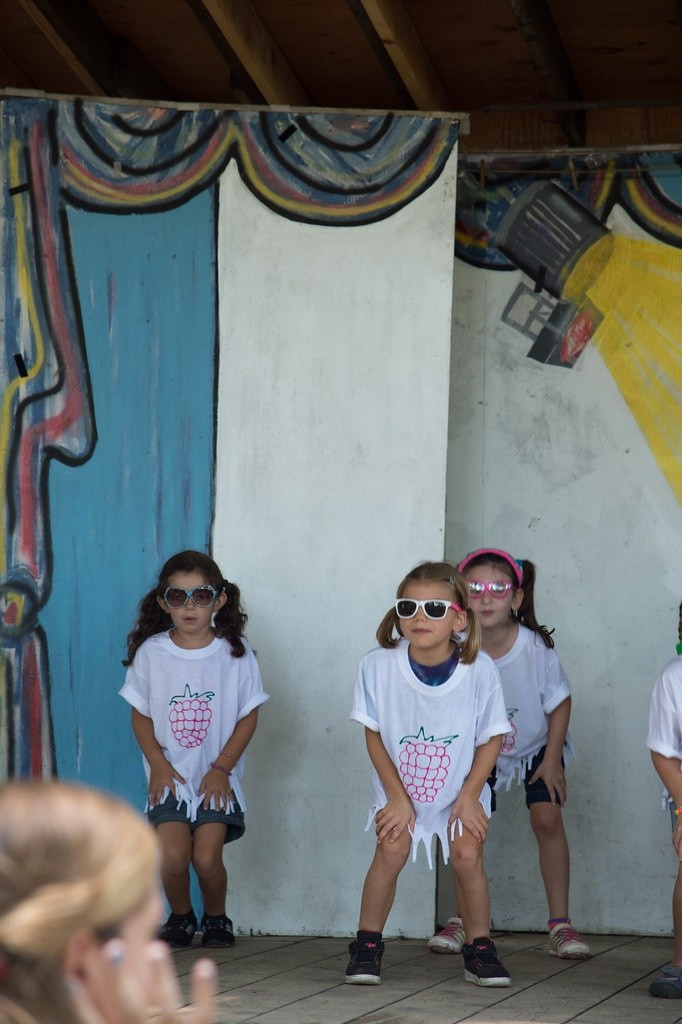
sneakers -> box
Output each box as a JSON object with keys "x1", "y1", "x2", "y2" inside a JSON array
[
  {"x1": 462, "y1": 936, "x2": 511, "y2": 987},
  {"x1": 547, "y1": 920, "x2": 593, "y2": 959},
  {"x1": 649, "y1": 963, "x2": 682, "y2": 999},
  {"x1": 345, "y1": 930, "x2": 385, "y2": 984},
  {"x1": 427, "y1": 915, "x2": 466, "y2": 953},
  {"x1": 200, "y1": 912, "x2": 236, "y2": 947},
  {"x1": 160, "y1": 909, "x2": 197, "y2": 947}
]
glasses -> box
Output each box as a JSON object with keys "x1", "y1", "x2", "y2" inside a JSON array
[
  {"x1": 396, "y1": 597, "x2": 463, "y2": 621},
  {"x1": 160, "y1": 584, "x2": 220, "y2": 608},
  {"x1": 466, "y1": 578, "x2": 518, "y2": 598}
]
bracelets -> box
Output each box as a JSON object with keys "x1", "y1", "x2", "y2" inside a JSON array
[
  {"x1": 211, "y1": 763, "x2": 233, "y2": 776},
  {"x1": 221, "y1": 751, "x2": 237, "y2": 763}
]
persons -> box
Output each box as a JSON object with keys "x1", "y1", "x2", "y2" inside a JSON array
[
  {"x1": 427, "y1": 549, "x2": 591, "y2": 960},
  {"x1": 118, "y1": 550, "x2": 270, "y2": 946},
  {"x1": 645, "y1": 600, "x2": 682, "y2": 999},
  {"x1": 0, "y1": 778, "x2": 215, "y2": 1024},
  {"x1": 342, "y1": 562, "x2": 512, "y2": 988}
]
